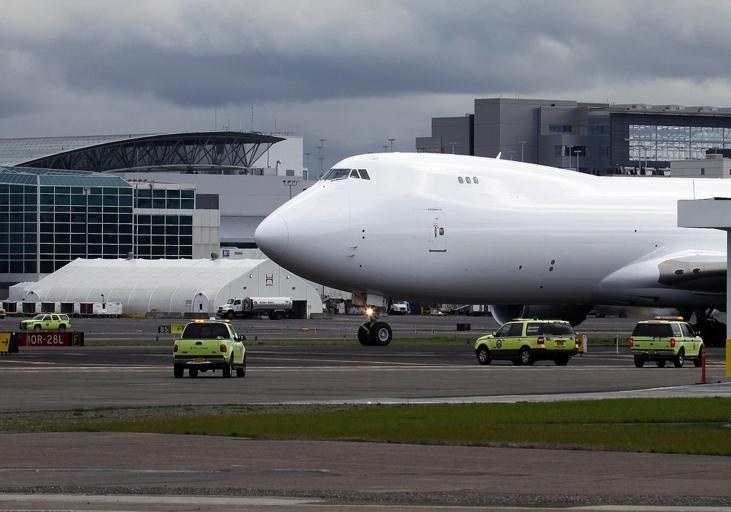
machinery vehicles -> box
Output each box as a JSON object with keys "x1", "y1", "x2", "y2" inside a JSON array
[{"x1": 216, "y1": 296, "x2": 295, "y2": 320}]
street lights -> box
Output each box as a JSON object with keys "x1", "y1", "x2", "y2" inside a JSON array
[
  {"x1": 519, "y1": 141, "x2": 527, "y2": 161},
  {"x1": 276, "y1": 160, "x2": 281, "y2": 176},
  {"x1": 450, "y1": 142, "x2": 457, "y2": 153},
  {"x1": 574, "y1": 151, "x2": 583, "y2": 171},
  {"x1": 388, "y1": 139, "x2": 395, "y2": 152},
  {"x1": 281, "y1": 178, "x2": 297, "y2": 200},
  {"x1": 306, "y1": 139, "x2": 328, "y2": 175},
  {"x1": 568, "y1": 145, "x2": 575, "y2": 167},
  {"x1": 383, "y1": 145, "x2": 389, "y2": 152},
  {"x1": 507, "y1": 151, "x2": 517, "y2": 161}
]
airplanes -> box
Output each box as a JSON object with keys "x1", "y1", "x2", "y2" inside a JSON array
[{"x1": 251, "y1": 151, "x2": 731, "y2": 349}]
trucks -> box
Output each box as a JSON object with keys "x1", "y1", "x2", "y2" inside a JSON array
[{"x1": 385, "y1": 299, "x2": 408, "y2": 316}]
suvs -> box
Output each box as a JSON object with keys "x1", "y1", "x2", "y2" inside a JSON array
[
  {"x1": 474, "y1": 316, "x2": 580, "y2": 367},
  {"x1": 19, "y1": 312, "x2": 71, "y2": 333},
  {"x1": 170, "y1": 316, "x2": 248, "y2": 379},
  {"x1": 628, "y1": 315, "x2": 706, "y2": 369}
]
